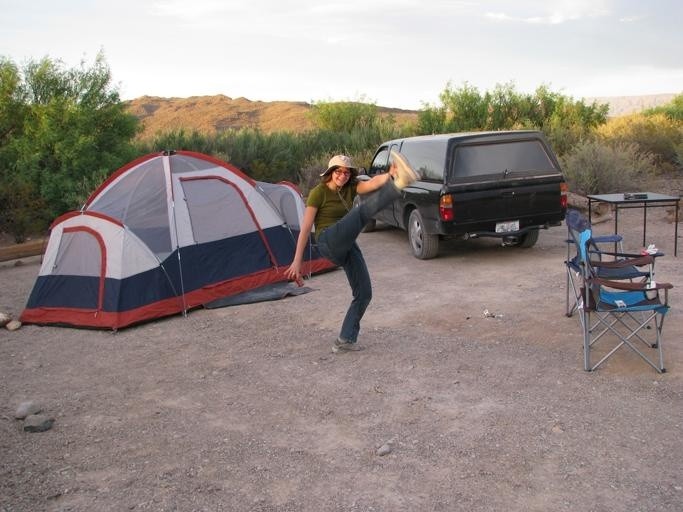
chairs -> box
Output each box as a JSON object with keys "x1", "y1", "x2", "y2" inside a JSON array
[{"x1": 563, "y1": 208, "x2": 673, "y2": 373}]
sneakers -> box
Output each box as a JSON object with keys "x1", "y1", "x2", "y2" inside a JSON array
[
  {"x1": 332, "y1": 337, "x2": 364, "y2": 353},
  {"x1": 391, "y1": 149, "x2": 420, "y2": 185}
]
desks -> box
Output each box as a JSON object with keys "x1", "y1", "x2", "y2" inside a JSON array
[{"x1": 584, "y1": 189, "x2": 680, "y2": 260}]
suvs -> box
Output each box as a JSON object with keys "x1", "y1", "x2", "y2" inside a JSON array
[{"x1": 352, "y1": 128, "x2": 569, "y2": 260}]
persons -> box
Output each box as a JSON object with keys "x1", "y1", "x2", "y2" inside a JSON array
[{"x1": 282, "y1": 147, "x2": 420, "y2": 354}]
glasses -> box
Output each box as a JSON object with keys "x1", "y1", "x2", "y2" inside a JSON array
[{"x1": 334, "y1": 168, "x2": 351, "y2": 176}]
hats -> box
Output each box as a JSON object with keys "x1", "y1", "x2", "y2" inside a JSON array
[{"x1": 319, "y1": 155, "x2": 359, "y2": 177}]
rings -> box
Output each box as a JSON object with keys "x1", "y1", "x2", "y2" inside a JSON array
[{"x1": 291, "y1": 273, "x2": 294, "y2": 275}]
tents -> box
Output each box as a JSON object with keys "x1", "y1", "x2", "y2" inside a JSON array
[{"x1": 19, "y1": 149, "x2": 337, "y2": 331}]
